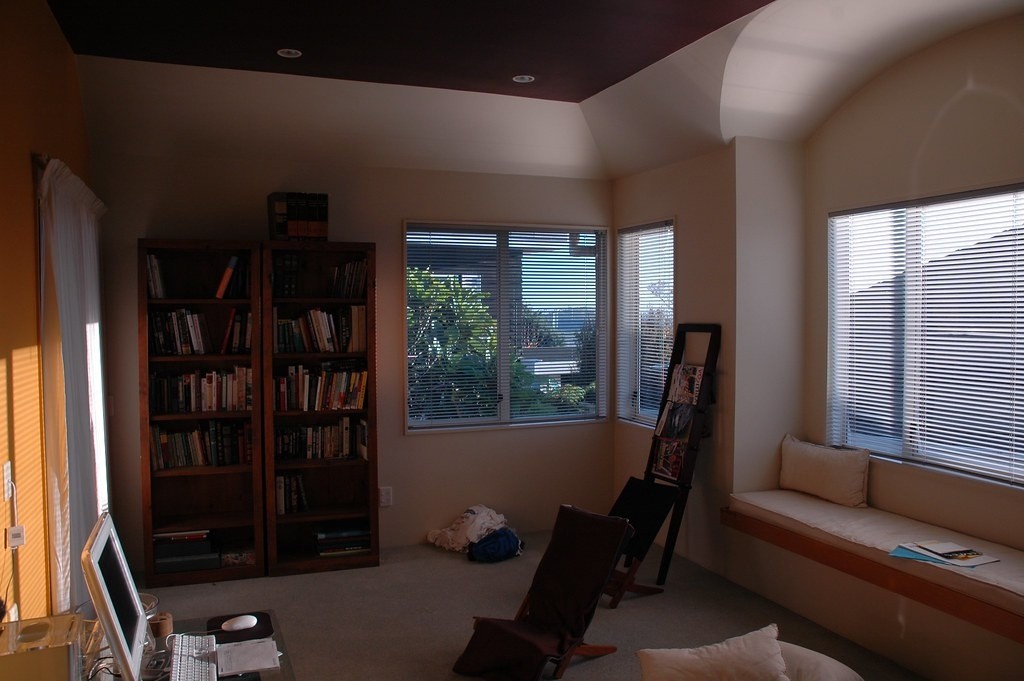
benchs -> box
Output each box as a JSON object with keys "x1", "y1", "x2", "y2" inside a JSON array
[{"x1": 719, "y1": 489, "x2": 1023, "y2": 681}]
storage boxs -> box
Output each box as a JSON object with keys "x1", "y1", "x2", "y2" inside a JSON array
[
  {"x1": 154, "y1": 541, "x2": 211, "y2": 557},
  {"x1": 0, "y1": 614, "x2": 87, "y2": 681},
  {"x1": 155, "y1": 548, "x2": 219, "y2": 572},
  {"x1": 221, "y1": 546, "x2": 256, "y2": 567}
]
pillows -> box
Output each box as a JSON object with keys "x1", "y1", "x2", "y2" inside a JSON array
[
  {"x1": 639, "y1": 623, "x2": 789, "y2": 681},
  {"x1": 780, "y1": 435, "x2": 869, "y2": 508},
  {"x1": 775, "y1": 640, "x2": 865, "y2": 681}
]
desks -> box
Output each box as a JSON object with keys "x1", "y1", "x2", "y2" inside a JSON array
[{"x1": 114, "y1": 608, "x2": 297, "y2": 681}]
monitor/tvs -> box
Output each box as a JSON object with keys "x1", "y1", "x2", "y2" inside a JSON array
[{"x1": 81, "y1": 513, "x2": 147, "y2": 681}]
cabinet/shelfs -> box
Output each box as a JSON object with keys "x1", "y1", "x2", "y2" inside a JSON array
[{"x1": 138, "y1": 238, "x2": 380, "y2": 588}]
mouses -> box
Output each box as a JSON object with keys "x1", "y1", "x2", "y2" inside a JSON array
[{"x1": 221, "y1": 615, "x2": 257, "y2": 631}]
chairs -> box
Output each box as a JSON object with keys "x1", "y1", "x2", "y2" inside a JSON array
[{"x1": 453, "y1": 504, "x2": 636, "y2": 681}]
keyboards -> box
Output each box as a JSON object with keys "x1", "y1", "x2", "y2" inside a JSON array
[{"x1": 170, "y1": 634, "x2": 217, "y2": 681}]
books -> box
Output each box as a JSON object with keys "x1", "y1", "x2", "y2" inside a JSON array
[
  {"x1": 215, "y1": 637, "x2": 280, "y2": 677},
  {"x1": 272, "y1": 359, "x2": 368, "y2": 411},
  {"x1": 273, "y1": 471, "x2": 309, "y2": 514},
  {"x1": 276, "y1": 305, "x2": 367, "y2": 354},
  {"x1": 146, "y1": 254, "x2": 167, "y2": 299},
  {"x1": 888, "y1": 540, "x2": 1000, "y2": 570},
  {"x1": 216, "y1": 253, "x2": 250, "y2": 299},
  {"x1": 327, "y1": 258, "x2": 368, "y2": 298},
  {"x1": 273, "y1": 416, "x2": 368, "y2": 462},
  {"x1": 149, "y1": 365, "x2": 253, "y2": 416},
  {"x1": 148, "y1": 420, "x2": 250, "y2": 472},
  {"x1": 148, "y1": 308, "x2": 251, "y2": 357},
  {"x1": 314, "y1": 528, "x2": 370, "y2": 557},
  {"x1": 152, "y1": 529, "x2": 257, "y2": 574},
  {"x1": 650, "y1": 364, "x2": 704, "y2": 481},
  {"x1": 273, "y1": 253, "x2": 297, "y2": 298}
]
